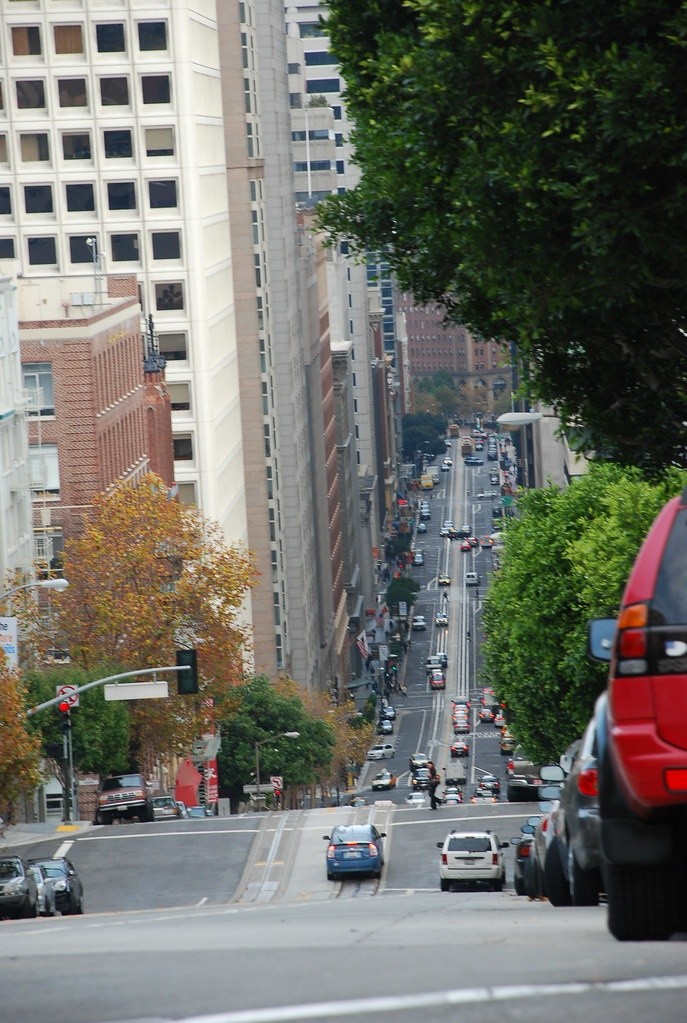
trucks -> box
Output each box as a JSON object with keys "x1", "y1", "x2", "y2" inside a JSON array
[
  {"x1": 506, "y1": 744, "x2": 560, "y2": 802},
  {"x1": 442, "y1": 763, "x2": 468, "y2": 786},
  {"x1": 420, "y1": 466, "x2": 439, "y2": 490}
]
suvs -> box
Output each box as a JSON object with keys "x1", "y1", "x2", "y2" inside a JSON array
[
  {"x1": 436, "y1": 829, "x2": 510, "y2": 892},
  {"x1": 94, "y1": 774, "x2": 155, "y2": 824}
]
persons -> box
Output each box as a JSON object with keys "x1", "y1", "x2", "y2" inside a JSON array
[
  {"x1": 429, "y1": 492, "x2": 434, "y2": 502},
  {"x1": 408, "y1": 638, "x2": 412, "y2": 651},
  {"x1": 403, "y1": 639, "x2": 407, "y2": 654},
  {"x1": 475, "y1": 588, "x2": 479, "y2": 599},
  {"x1": 465, "y1": 628, "x2": 472, "y2": 642},
  {"x1": 299, "y1": 798, "x2": 305, "y2": 809},
  {"x1": 441, "y1": 590, "x2": 450, "y2": 602},
  {"x1": 427, "y1": 760, "x2": 447, "y2": 810},
  {"x1": 400, "y1": 683, "x2": 408, "y2": 700},
  {"x1": 467, "y1": 489, "x2": 472, "y2": 496}
]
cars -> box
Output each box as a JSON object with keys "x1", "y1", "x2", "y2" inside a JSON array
[
  {"x1": 539, "y1": 717, "x2": 607, "y2": 907},
  {"x1": 188, "y1": 807, "x2": 213, "y2": 818},
  {"x1": 587, "y1": 493, "x2": 687, "y2": 942},
  {"x1": 0, "y1": 855, "x2": 84, "y2": 919},
  {"x1": 441, "y1": 429, "x2": 499, "y2": 485},
  {"x1": 345, "y1": 744, "x2": 430, "y2": 807},
  {"x1": 442, "y1": 775, "x2": 502, "y2": 804},
  {"x1": 323, "y1": 823, "x2": 387, "y2": 881},
  {"x1": 377, "y1": 490, "x2": 517, "y2": 757},
  {"x1": 510, "y1": 738, "x2": 582, "y2": 908},
  {"x1": 152, "y1": 796, "x2": 192, "y2": 822}
]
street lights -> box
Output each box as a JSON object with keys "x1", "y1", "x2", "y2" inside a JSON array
[
  {"x1": 255, "y1": 732, "x2": 300, "y2": 812},
  {"x1": 416, "y1": 441, "x2": 430, "y2": 451}
]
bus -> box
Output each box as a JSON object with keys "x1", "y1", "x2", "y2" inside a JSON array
[{"x1": 449, "y1": 424, "x2": 473, "y2": 456}]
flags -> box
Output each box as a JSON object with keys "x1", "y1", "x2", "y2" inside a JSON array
[
  {"x1": 355, "y1": 629, "x2": 373, "y2": 659},
  {"x1": 375, "y1": 606, "x2": 389, "y2": 627}
]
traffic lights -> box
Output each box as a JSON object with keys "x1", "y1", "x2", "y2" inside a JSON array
[{"x1": 58, "y1": 700, "x2": 72, "y2": 733}]
